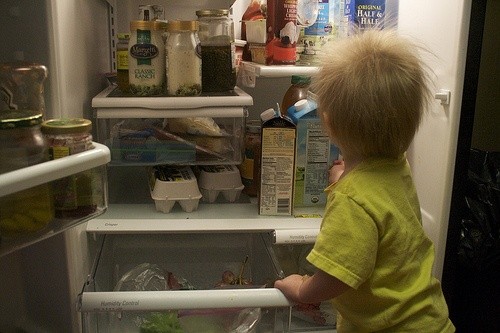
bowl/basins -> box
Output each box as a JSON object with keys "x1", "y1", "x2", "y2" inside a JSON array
[{"x1": 240, "y1": 18, "x2": 308, "y2": 65}]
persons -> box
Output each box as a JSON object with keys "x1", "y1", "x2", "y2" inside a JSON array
[{"x1": 274, "y1": 8, "x2": 456, "y2": 333}]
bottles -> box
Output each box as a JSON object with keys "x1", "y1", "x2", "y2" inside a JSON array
[
  {"x1": 281, "y1": 75, "x2": 312, "y2": 116},
  {"x1": 115, "y1": 2, "x2": 237, "y2": 96}
]
